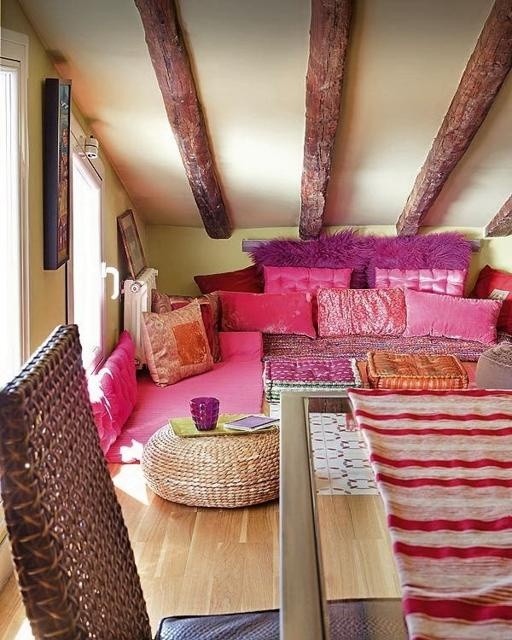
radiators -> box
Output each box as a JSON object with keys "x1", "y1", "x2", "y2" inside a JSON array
[{"x1": 122, "y1": 269, "x2": 163, "y2": 372}]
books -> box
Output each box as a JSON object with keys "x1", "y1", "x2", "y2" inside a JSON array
[{"x1": 225, "y1": 415, "x2": 280, "y2": 432}]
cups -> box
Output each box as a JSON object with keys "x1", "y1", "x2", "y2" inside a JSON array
[{"x1": 190, "y1": 396, "x2": 220, "y2": 431}]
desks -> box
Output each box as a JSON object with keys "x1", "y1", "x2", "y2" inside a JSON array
[{"x1": 272, "y1": 384, "x2": 512, "y2": 639}]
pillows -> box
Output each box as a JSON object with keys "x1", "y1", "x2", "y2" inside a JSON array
[
  {"x1": 468, "y1": 265, "x2": 511, "y2": 328},
  {"x1": 403, "y1": 289, "x2": 503, "y2": 345},
  {"x1": 218, "y1": 292, "x2": 317, "y2": 339},
  {"x1": 141, "y1": 287, "x2": 220, "y2": 387},
  {"x1": 315, "y1": 288, "x2": 406, "y2": 338},
  {"x1": 264, "y1": 266, "x2": 351, "y2": 293},
  {"x1": 374, "y1": 266, "x2": 467, "y2": 298},
  {"x1": 194, "y1": 265, "x2": 262, "y2": 296}
]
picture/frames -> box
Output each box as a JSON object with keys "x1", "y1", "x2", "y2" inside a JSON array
[
  {"x1": 45, "y1": 74, "x2": 73, "y2": 271},
  {"x1": 117, "y1": 208, "x2": 148, "y2": 278}
]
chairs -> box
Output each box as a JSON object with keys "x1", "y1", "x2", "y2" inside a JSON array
[{"x1": 2, "y1": 319, "x2": 405, "y2": 640}]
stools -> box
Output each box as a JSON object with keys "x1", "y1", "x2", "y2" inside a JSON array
[{"x1": 145, "y1": 419, "x2": 284, "y2": 507}]
